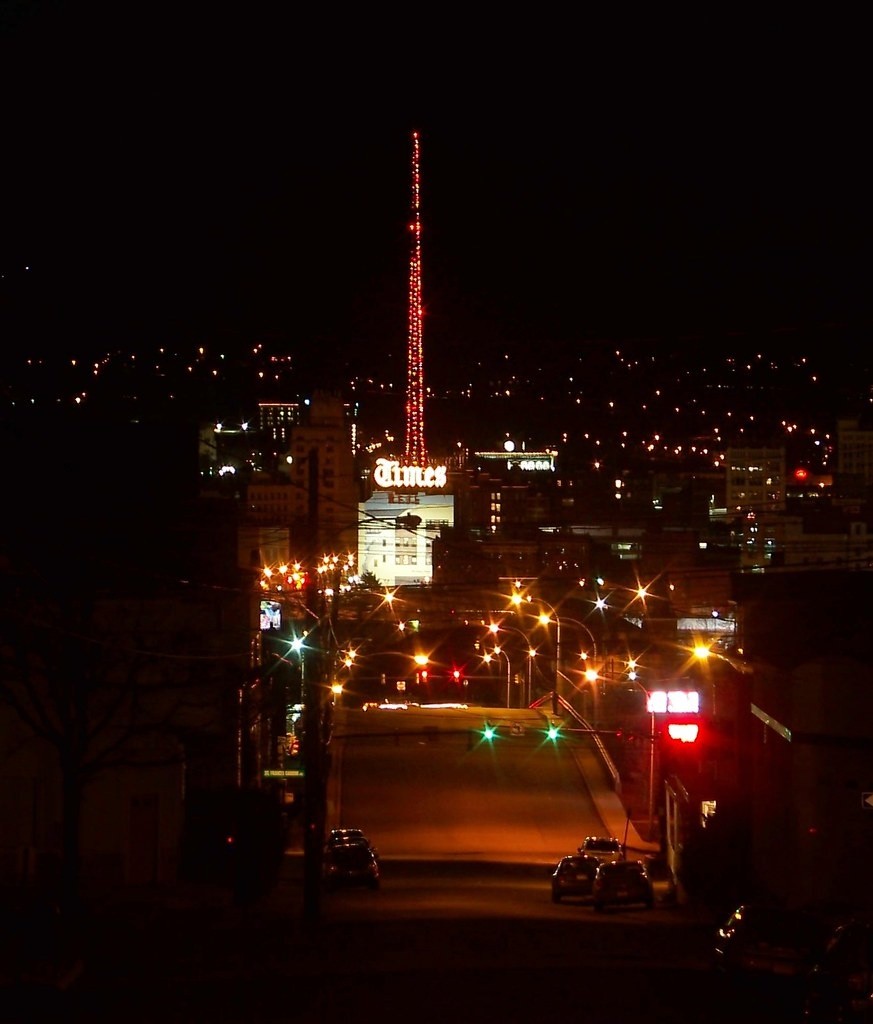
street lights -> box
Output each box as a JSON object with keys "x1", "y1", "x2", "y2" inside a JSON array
[
  {"x1": 587, "y1": 670, "x2": 655, "y2": 840},
  {"x1": 512, "y1": 597, "x2": 560, "y2": 715},
  {"x1": 307, "y1": 515, "x2": 422, "y2": 919},
  {"x1": 491, "y1": 625, "x2": 532, "y2": 705},
  {"x1": 541, "y1": 615, "x2": 597, "y2": 724},
  {"x1": 496, "y1": 648, "x2": 510, "y2": 708}
]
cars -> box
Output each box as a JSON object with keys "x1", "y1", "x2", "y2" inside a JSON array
[
  {"x1": 552, "y1": 856, "x2": 600, "y2": 903},
  {"x1": 322, "y1": 844, "x2": 381, "y2": 894},
  {"x1": 326, "y1": 829, "x2": 380, "y2": 865},
  {"x1": 592, "y1": 860, "x2": 654, "y2": 914},
  {"x1": 579, "y1": 836, "x2": 624, "y2": 865}
]
